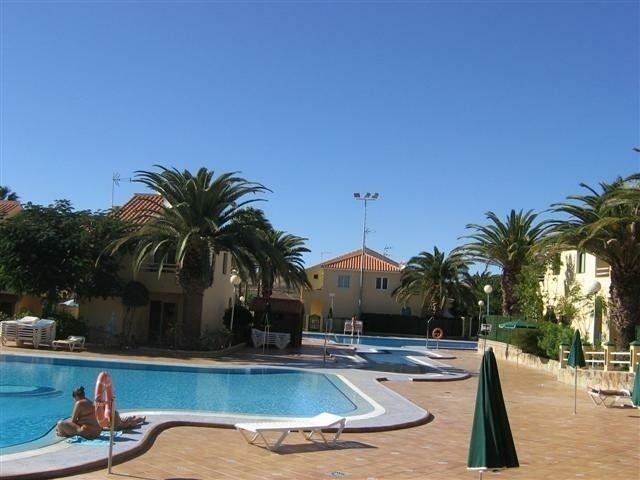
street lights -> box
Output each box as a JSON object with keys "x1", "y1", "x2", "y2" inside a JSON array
[
  {"x1": 228, "y1": 269, "x2": 241, "y2": 347},
  {"x1": 353, "y1": 192, "x2": 379, "y2": 318},
  {"x1": 469, "y1": 285, "x2": 493, "y2": 336}
]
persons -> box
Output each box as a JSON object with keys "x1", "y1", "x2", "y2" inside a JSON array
[
  {"x1": 102, "y1": 396, "x2": 146, "y2": 428},
  {"x1": 56, "y1": 386, "x2": 101, "y2": 440}
]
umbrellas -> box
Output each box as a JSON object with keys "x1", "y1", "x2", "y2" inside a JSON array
[
  {"x1": 262, "y1": 301, "x2": 271, "y2": 354},
  {"x1": 631, "y1": 360, "x2": 640, "y2": 409},
  {"x1": 567, "y1": 329, "x2": 586, "y2": 415},
  {"x1": 465, "y1": 347, "x2": 519, "y2": 479},
  {"x1": 498, "y1": 319, "x2": 538, "y2": 367}
]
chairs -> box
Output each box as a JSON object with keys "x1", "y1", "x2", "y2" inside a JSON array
[
  {"x1": 1, "y1": 320, "x2": 56, "y2": 348},
  {"x1": 588, "y1": 389, "x2": 636, "y2": 408},
  {"x1": 52, "y1": 335, "x2": 85, "y2": 351},
  {"x1": 235, "y1": 411, "x2": 346, "y2": 452},
  {"x1": 251, "y1": 329, "x2": 290, "y2": 349}
]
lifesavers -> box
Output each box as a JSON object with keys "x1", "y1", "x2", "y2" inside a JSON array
[
  {"x1": 432, "y1": 327, "x2": 443, "y2": 339},
  {"x1": 93, "y1": 370, "x2": 114, "y2": 428}
]
